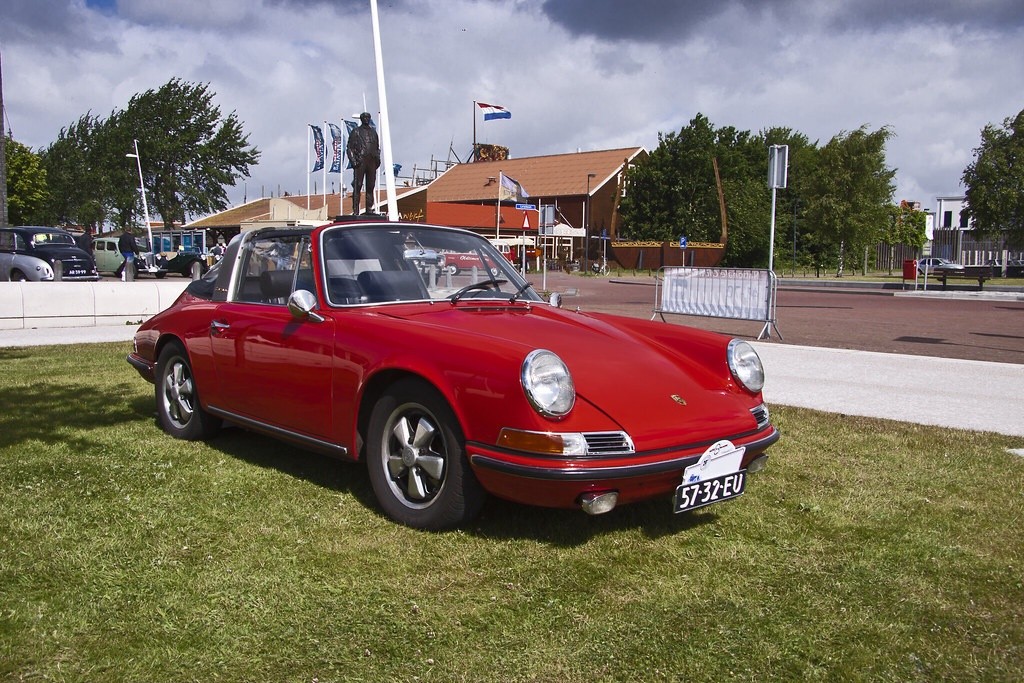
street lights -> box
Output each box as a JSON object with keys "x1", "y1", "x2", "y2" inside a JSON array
[
  {"x1": 585, "y1": 173, "x2": 596, "y2": 275},
  {"x1": 126, "y1": 154, "x2": 154, "y2": 252}
]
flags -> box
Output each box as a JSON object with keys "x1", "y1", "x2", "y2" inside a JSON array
[
  {"x1": 329, "y1": 124, "x2": 345, "y2": 173},
  {"x1": 344, "y1": 121, "x2": 358, "y2": 169},
  {"x1": 477, "y1": 103, "x2": 511, "y2": 121},
  {"x1": 500, "y1": 175, "x2": 529, "y2": 201},
  {"x1": 383, "y1": 163, "x2": 402, "y2": 177},
  {"x1": 310, "y1": 125, "x2": 327, "y2": 172}
]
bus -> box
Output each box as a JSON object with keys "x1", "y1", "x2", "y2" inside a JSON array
[{"x1": 441, "y1": 241, "x2": 513, "y2": 277}]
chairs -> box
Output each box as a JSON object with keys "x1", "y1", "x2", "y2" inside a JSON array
[
  {"x1": 357, "y1": 269, "x2": 421, "y2": 303},
  {"x1": 261, "y1": 269, "x2": 312, "y2": 305}
]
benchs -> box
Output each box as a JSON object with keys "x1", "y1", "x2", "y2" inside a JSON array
[
  {"x1": 932, "y1": 266, "x2": 993, "y2": 291},
  {"x1": 237, "y1": 274, "x2": 361, "y2": 303}
]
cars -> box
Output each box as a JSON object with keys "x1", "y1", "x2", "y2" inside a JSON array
[
  {"x1": 91, "y1": 237, "x2": 169, "y2": 279},
  {"x1": 153, "y1": 236, "x2": 207, "y2": 278},
  {"x1": 0, "y1": 226, "x2": 101, "y2": 283},
  {"x1": 985, "y1": 260, "x2": 1024, "y2": 266},
  {"x1": 918, "y1": 258, "x2": 964, "y2": 276},
  {"x1": 126, "y1": 223, "x2": 780, "y2": 531},
  {"x1": 403, "y1": 242, "x2": 442, "y2": 285}
]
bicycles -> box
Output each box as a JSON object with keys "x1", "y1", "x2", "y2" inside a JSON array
[{"x1": 592, "y1": 258, "x2": 610, "y2": 277}]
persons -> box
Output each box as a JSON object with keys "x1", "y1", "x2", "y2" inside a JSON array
[
  {"x1": 77, "y1": 229, "x2": 93, "y2": 255},
  {"x1": 510, "y1": 247, "x2": 515, "y2": 261},
  {"x1": 115, "y1": 226, "x2": 141, "y2": 278},
  {"x1": 347, "y1": 112, "x2": 381, "y2": 216},
  {"x1": 210, "y1": 243, "x2": 227, "y2": 264},
  {"x1": 520, "y1": 247, "x2": 526, "y2": 271}
]
